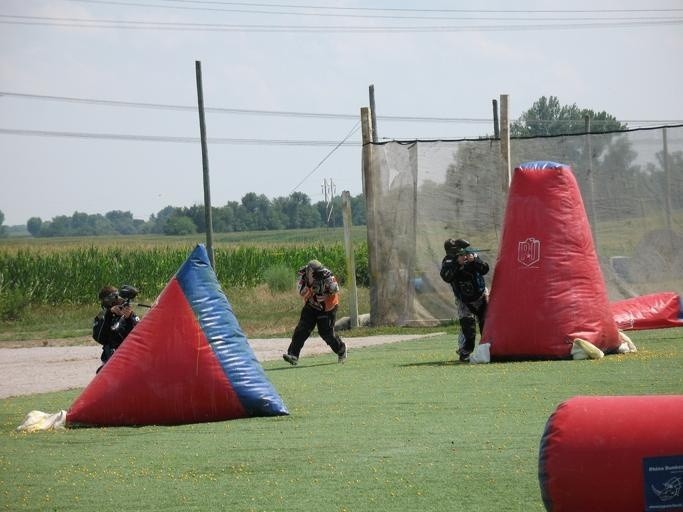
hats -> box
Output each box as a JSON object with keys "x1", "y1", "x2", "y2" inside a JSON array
[
  {"x1": 308, "y1": 260, "x2": 324, "y2": 272},
  {"x1": 444, "y1": 239, "x2": 456, "y2": 248}
]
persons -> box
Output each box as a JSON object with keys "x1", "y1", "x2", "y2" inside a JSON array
[
  {"x1": 90, "y1": 284, "x2": 142, "y2": 376},
  {"x1": 438, "y1": 237, "x2": 490, "y2": 363},
  {"x1": 281, "y1": 258, "x2": 347, "y2": 366}
]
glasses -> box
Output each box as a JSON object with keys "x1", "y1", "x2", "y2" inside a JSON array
[{"x1": 106, "y1": 292, "x2": 119, "y2": 297}]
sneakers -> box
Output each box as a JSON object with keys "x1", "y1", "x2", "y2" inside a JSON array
[
  {"x1": 338, "y1": 344, "x2": 347, "y2": 362},
  {"x1": 460, "y1": 355, "x2": 470, "y2": 361},
  {"x1": 283, "y1": 354, "x2": 298, "y2": 365}
]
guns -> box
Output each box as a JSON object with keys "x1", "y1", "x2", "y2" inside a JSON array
[
  {"x1": 311, "y1": 270, "x2": 334, "y2": 296},
  {"x1": 448, "y1": 238, "x2": 490, "y2": 261},
  {"x1": 111, "y1": 285, "x2": 152, "y2": 324}
]
